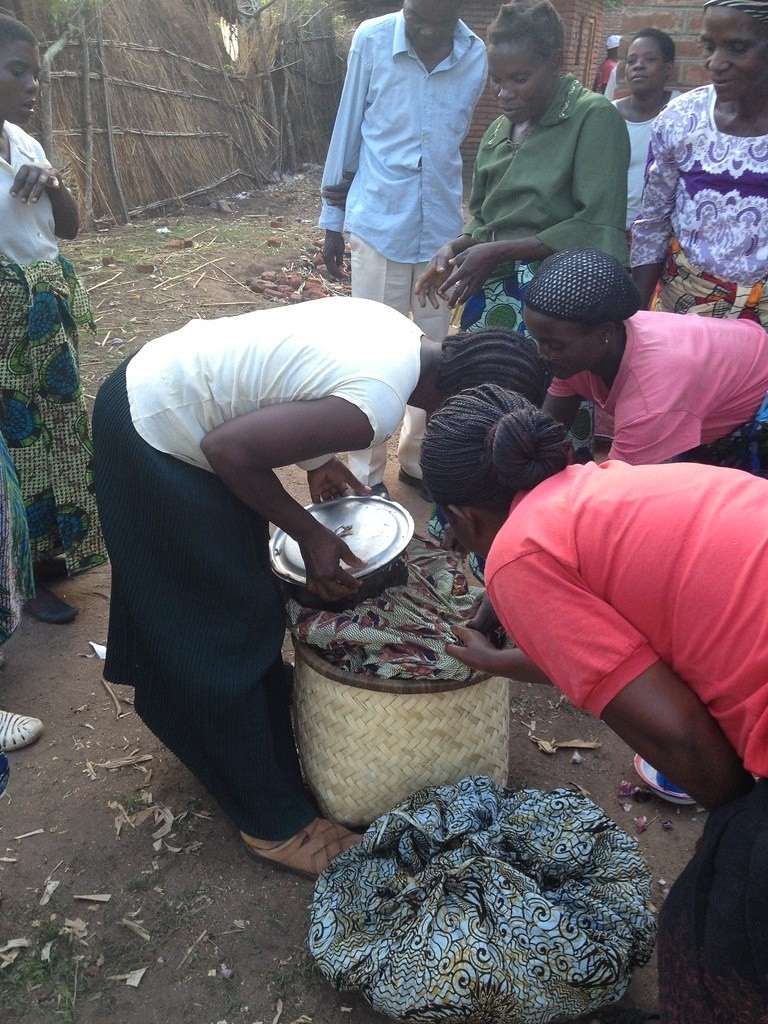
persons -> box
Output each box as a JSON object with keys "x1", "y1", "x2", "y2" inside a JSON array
[
  {"x1": 0, "y1": 14, "x2": 106, "y2": 624},
  {"x1": 412, "y1": 0, "x2": 634, "y2": 547},
  {"x1": 436, "y1": 248, "x2": 767, "y2": 640},
  {"x1": 607, "y1": 26, "x2": 689, "y2": 249},
  {"x1": 418, "y1": 382, "x2": 767, "y2": 1024},
  {"x1": 316, "y1": 0, "x2": 489, "y2": 502},
  {"x1": 91, "y1": 293, "x2": 547, "y2": 881},
  {"x1": 0, "y1": 429, "x2": 43, "y2": 757},
  {"x1": 591, "y1": 34, "x2": 622, "y2": 94},
  {"x1": 628, "y1": 0, "x2": 767, "y2": 330}
]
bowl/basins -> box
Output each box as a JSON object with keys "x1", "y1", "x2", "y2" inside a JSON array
[{"x1": 634, "y1": 753, "x2": 697, "y2": 805}]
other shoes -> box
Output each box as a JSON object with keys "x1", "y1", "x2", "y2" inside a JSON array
[
  {"x1": 0, "y1": 711, "x2": 44, "y2": 750},
  {"x1": 25, "y1": 589, "x2": 79, "y2": 622},
  {"x1": 246, "y1": 817, "x2": 364, "y2": 882},
  {"x1": 38, "y1": 555, "x2": 68, "y2": 581},
  {"x1": 398, "y1": 466, "x2": 432, "y2": 502},
  {"x1": 366, "y1": 483, "x2": 388, "y2": 501}
]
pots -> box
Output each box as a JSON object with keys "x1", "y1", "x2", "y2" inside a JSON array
[{"x1": 268, "y1": 494, "x2": 415, "y2": 612}]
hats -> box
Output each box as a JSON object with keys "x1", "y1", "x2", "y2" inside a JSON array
[
  {"x1": 607, "y1": 35, "x2": 621, "y2": 49},
  {"x1": 703, "y1": 0, "x2": 768, "y2": 26}
]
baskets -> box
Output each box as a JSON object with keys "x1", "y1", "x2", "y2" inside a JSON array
[{"x1": 290, "y1": 629, "x2": 510, "y2": 830}]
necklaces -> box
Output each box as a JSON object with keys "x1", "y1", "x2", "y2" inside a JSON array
[{"x1": 506, "y1": 124, "x2": 538, "y2": 156}]
